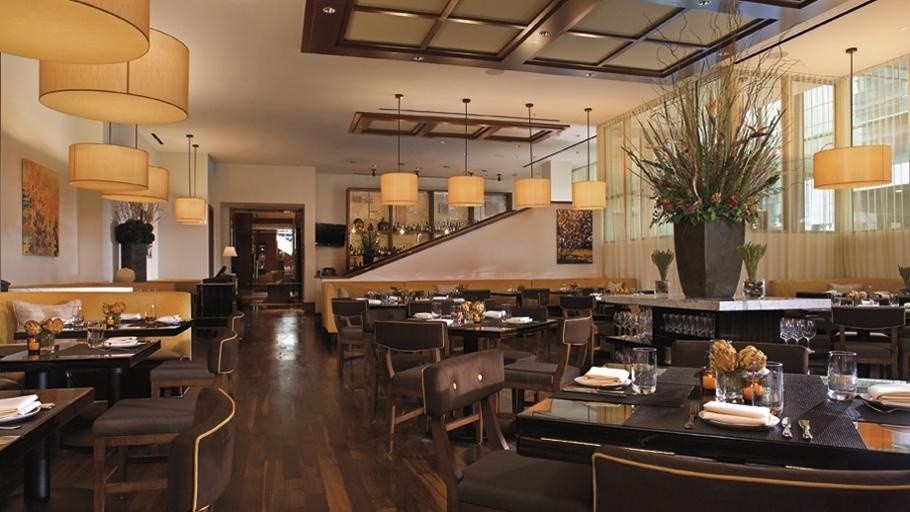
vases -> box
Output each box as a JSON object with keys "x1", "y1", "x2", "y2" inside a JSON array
[
  {"x1": 671, "y1": 210, "x2": 745, "y2": 300},
  {"x1": 122, "y1": 238, "x2": 152, "y2": 281}
]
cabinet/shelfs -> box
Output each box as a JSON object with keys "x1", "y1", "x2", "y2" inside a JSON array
[{"x1": 343, "y1": 185, "x2": 515, "y2": 274}]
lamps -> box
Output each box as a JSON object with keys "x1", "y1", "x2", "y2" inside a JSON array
[
  {"x1": 63, "y1": 120, "x2": 152, "y2": 194},
  {"x1": 448, "y1": 97, "x2": 490, "y2": 209},
  {"x1": 572, "y1": 107, "x2": 609, "y2": 212},
  {"x1": 34, "y1": 21, "x2": 191, "y2": 129},
  {"x1": 380, "y1": 91, "x2": 420, "y2": 206},
  {"x1": 515, "y1": 101, "x2": 553, "y2": 207},
  {"x1": 180, "y1": 141, "x2": 215, "y2": 223},
  {"x1": 102, "y1": 126, "x2": 173, "y2": 205},
  {"x1": 171, "y1": 131, "x2": 210, "y2": 225},
  {"x1": 812, "y1": 46, "x2": 893, "y2": 194},
  {"x1": 223, "y1": 245, "x2": 239, "y2": 274},
  {"x1": 0, "y1": 0, "x2": 155, "y2": 65}
]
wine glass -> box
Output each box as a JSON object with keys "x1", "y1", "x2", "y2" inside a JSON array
[
  {"x1": 612, "y1": 310, "x2": 652, "y2": 341},
  {"x1": 779, "y1": 317, "x2": 817, "y2": 354}
]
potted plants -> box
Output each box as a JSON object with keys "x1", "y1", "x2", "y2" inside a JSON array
[
  {"x1": 742, "y1": 242, "x2": 766, "y2": 303},
  {"x1": 647, "y1": 249, "x2": 674, "y2": 294}
]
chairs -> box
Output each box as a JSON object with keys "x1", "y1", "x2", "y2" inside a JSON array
[
  {"x1": 420, "y1": 346, "x2": 596, "y2": 512},
  {"x1": 318, "y1": 272, "x2": 910, "y2": 454},
  {"x1": 587, "y1": 446, "x2": 910, "y2": 512},
  {"x1": 90, "y1": 308, "x2": 249, "y2": 512}
]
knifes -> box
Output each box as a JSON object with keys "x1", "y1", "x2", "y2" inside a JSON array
[
  {"x1": 781, "y1": 416, "x2": 794, "y2": 439},
  {"x1": 798, "y1": 419, "x2": 813, "y2": 439},
  {"x1": 561, "y1": 384, "x2": 627, "y2": 397}
]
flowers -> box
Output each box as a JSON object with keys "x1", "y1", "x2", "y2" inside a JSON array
[
  {"x1": 625, "y1": 3, "x2": 793, "y2": 230},
  {"x1": 109, "y1": 198, "x2": 168, "y2": 245}
]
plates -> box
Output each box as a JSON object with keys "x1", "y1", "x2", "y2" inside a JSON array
[
  {"x1": 573, "y1": 375, "x2": 631, "y2": 388},
  {"x1": 158, "y1": 320, "x2": 180, "y2": 324},
  {"x1": 105, "y1": 343, "x2": 138, "y2": 349},
  {"x1": 0, "y1": 405, "x2": 42, "y2": 424},
  {"x1": 486, "y1": 313, "x2": 500, "y2": 319},
  {"x1": 696, "y1": 410, "x2": 780, "y2": 432},
  {"x1": 860, "y1": 392, "x2": 910, "y2": 409},
  {"x1": 120, "y1": 317, "x2": 140, "y2": 322},
  {"x1": 505, "y1": 318, "x2": 532, "y2": 325},
  {"x1": 427, "y1": 318, "x2": 453, "y2": 326}
]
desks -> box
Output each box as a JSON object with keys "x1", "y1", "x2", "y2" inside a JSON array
[{"x1": 0, "y1": 314, "x2": 193, "y2": 511}]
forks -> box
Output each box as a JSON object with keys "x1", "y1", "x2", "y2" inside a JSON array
[
  {"x1": 866, "y1": 405, "x2": 910, "y2": 415},
  {"x1": 685, "y1": 399, "x2": 700, "y2": 429}
]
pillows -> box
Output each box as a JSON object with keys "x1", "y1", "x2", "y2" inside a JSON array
[{"x1": 11, "y1": 296, "x2": 84, "y2": 341}]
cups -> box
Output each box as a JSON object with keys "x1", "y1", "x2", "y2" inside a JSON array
[
  {"x1": 702, "y1": 370, "x2": 716, "y2": 395},
  {"x1": 432, "y1": 303, "x2": 442, "y2": 319},
  {"x1": 87, "y1": 320, "x2": 104, "y2": 349},
  {"x1": 828, "y1": 350, "x2": 858, "y2": 401},
  {"x1": 750, "y1": 360, "x2": 786, "y2": 414},
  {"x1": 368, "y1": 289, "x2": 394, "y2": 305},
  {"x1": 501, "y1": 303, "x2": 512, "y2": 323},
  {"x1": 743, "y1": 375, "x2": 752, "y2": 404},
  {"x1": 71, "y1": 305, "x2": 86, "y2": 326},
  {"x1": 144, "y1": 303, "x2": 156, "y2": 324},
  {"x1": 450, "y1": 306, "x2": 462, "y2": 327},
  {"x1": 630, "y1": 347, "x2": 657, "y2": 396}
]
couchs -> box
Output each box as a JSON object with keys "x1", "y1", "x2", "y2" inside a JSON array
[{"x1": 1, "y1": 288, "x2": 195, "y2": 382}]
